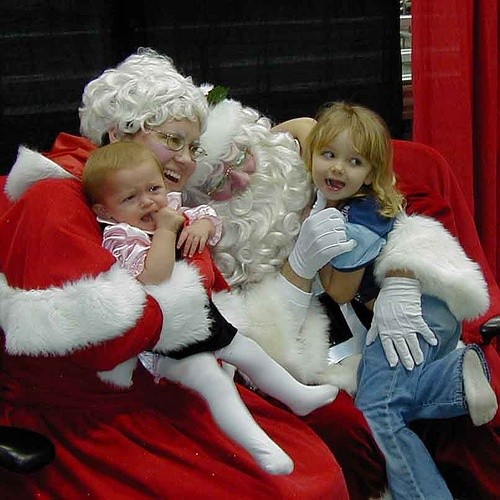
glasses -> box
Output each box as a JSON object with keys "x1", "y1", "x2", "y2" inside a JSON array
[
  {"x1": 207, "y1": 146, "x2": 248, "y2": 196},
  {"x1": 144, "y1": 126, "x2": 208, "y2": 162}
]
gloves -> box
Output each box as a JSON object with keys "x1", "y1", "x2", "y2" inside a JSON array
[
  {"x1": 288, "y1": 189, "x2": 357, "y2": 280},
  {"x1": 366, "y1": 277, "x2": 437, "y2": 371}
]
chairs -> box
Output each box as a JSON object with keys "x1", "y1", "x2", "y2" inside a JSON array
[{"x1": 0, "y1": 139, "x2": 500, "y2": 476}]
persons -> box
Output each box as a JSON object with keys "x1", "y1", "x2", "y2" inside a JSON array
[
  {"x1": 302, "y1": 102, "x2": 498, "y2": 500},
  {"x1": 0, "y1": 49, "x2": 349, "y2": 500},
  {"x1": 174, "y1": 85, "x2": 500, "y2": 500},
  {"x1": 81, "y1": 143, "x2": 339, "y2": 475}
]
